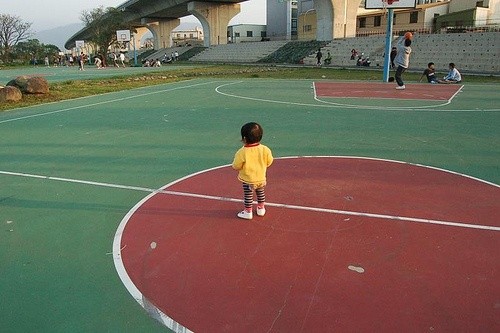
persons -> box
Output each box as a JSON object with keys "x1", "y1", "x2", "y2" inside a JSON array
[
  {"x1": 350, "y1": 48, "x2": 358, "y2": 60},
  {"x1": 118, "y1": 50, "x2": 127, "y2": 68},
  {"x1": 424, "y1": 60, "x2": 440, "y2": 86},
  {"x1": 227, "y1": 36, "x2": 232, "y2": 44},
  {"x1": 390, "y1": 46, "x2": 399, "y2": 68},
  {"x1": 442, "y1": 61, "x2": 462, "y2": 84},
  {"x1": 33, "y1": 56, "x2": 39, "y2": 69},
  {"x1": 323, "y1": 51, "x2": 333, "y2": 65},
  {"x1": 142, "y1": 57, "x2": 162, "y2": 68},
  {"x1": 163, "y1": 50, "x2": 180, "y2": 62},
  {"x1": 54, "y1": 52, "x2": 106, "y2": 70},
  {"x1": 44, "y1": 55, "x2": 51, "y2": 69},
  {"x1": 111, "y1": 51, "x2": 119, "y2": 69},
  {"x1": 315, "y1": 49, "x2": 323, "y2": 65},
  {"x1": 393, "y1": 35, "x2": 412, "y2": 90},
  {"x1": 233, "y1": 121, "x2": 274, "y2": 220}
]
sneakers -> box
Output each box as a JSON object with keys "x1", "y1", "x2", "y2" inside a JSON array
[
  {"x1": 396, "y1": 85, "x2": 406, "y2": 89},
  {"x1": 238, "y1": 210, "x2": 253, "y2": 220},
  {"x1": 257, "y1": 207, "x2": 266, "y2": 216},
  {"x1": 34, "y1": 65, "x2": 126, "y2": 71}
]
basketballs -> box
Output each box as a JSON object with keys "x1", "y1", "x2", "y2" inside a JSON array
[{"x1": 404, "y1": 32, "x2": 412, "y2": 39}]
